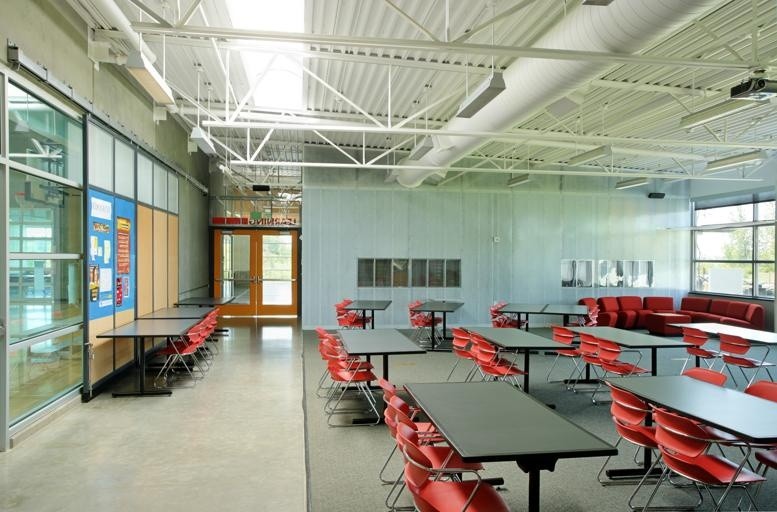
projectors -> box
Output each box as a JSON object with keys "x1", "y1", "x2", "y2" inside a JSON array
[{"x1": 730, "y1": 79, "x2": 777, "y2": 101}]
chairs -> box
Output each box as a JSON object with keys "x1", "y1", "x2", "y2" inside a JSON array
[{"x1": 151, "y1": 307, "x2": 220, "y2": 390}]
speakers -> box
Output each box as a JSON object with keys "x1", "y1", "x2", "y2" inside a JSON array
[
  {"x1": 253, "y1": 185, "x2": 270, "y2": 191},
  {"x1": 648, "y1": 193, "x2": 665, "y2": 199}
]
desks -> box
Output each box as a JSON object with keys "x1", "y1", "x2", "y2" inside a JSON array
[
  {"x1": 173, "y1": 296, "x2": 236, "y2": 341},
  {"x1": 138, "y1": 304, "x2": 217, "y2": 373},
  {"x1": 96, "y1": 319, "x2": 203, "y2": 396}
]
